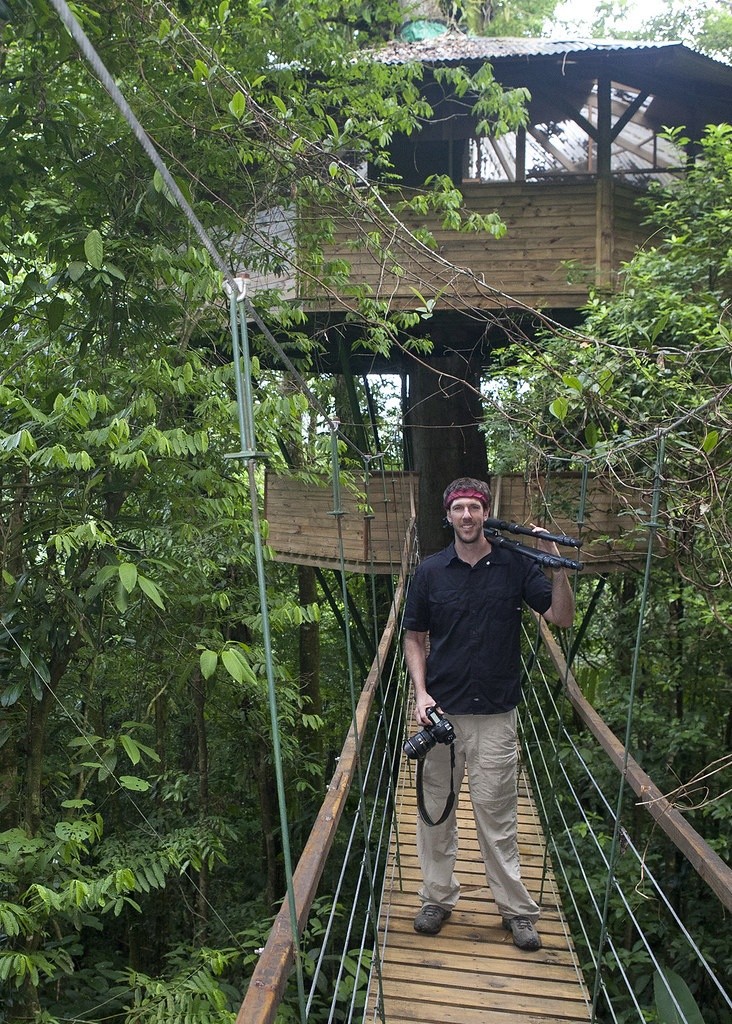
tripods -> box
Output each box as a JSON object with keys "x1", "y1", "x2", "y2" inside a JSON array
[{"x1": 483, "y1": 519, "x2": 584, "y2": 572}]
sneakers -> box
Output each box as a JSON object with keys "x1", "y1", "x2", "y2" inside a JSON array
[
  {"x1": 502, "y1": 916, "x2": 541, "y2": 950},
  {"x1": 414, "y1": 904, "x2": 451, "y2": 934}
]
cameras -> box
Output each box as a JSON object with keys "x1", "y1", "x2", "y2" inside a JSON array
[{"x1": 404, "y1": 706, "x2": 456, "y2": 760}]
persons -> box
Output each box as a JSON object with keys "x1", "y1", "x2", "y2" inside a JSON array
[{"x1": 404, "y1": 477, "x2": 575, "y2": 949}]
